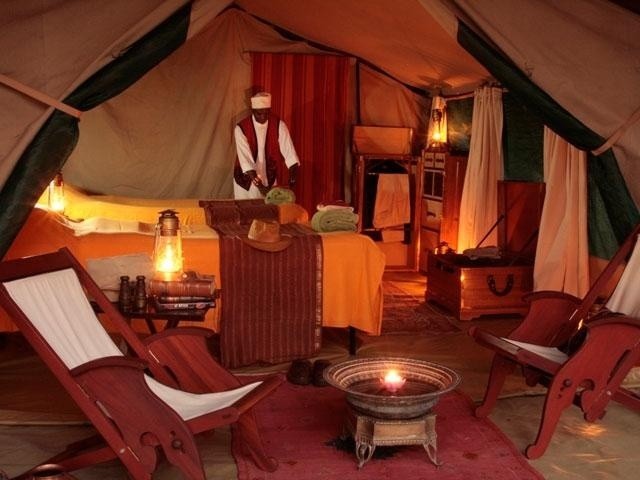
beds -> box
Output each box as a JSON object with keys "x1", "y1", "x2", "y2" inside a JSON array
[{"x1": 0, "y1": 177, "x2": 387, "y2": 358}]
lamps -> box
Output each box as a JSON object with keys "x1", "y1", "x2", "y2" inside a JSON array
[
  {"x1": 149, "y1": 209, "x2": 185, "y2": 283},
  {"x1": 48, "y1": 169, "x2": 65, "y2": 211},
  {"x1": 430, "y1": 106, "x2": 445, "y2": 148}
]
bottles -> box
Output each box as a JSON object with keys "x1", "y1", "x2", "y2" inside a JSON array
[{"x1": 119, "y1": 275, "x2": 148, "y2": 314}]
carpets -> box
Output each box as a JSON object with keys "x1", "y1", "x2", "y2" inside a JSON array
[
  {"x1": 227, "y1": 377, "x2": 545, "y2": 479},
  {"x1": 322, "y1": 279, "x2": 463, "y2": 339}
]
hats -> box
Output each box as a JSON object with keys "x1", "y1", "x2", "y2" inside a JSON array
[
  {"x1": 238, "y1": 217, "x2": 294, "y2": 253},
  {"x1": 250, "y1": 91, "x2": 272, "y2": 110}
]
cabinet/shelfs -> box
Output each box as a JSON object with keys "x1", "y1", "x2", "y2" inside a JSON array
[
  {"x1": 352, "y1": 153, "x2": 420, "y2": 275},
  {"x1": 418, "y1": 149, "x2": 472, "y2": 274}
]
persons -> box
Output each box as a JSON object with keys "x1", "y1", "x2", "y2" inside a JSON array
[{"x1": 233, "y1": 92, "x2": 301, "y2": 200}]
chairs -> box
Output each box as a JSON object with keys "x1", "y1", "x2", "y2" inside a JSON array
[
  {"x1": 1, "y1": 244, "x2": 282, "y2": 479},
  {"x1": 466, "y1": 224, "x2": 640, "y2": 462}
]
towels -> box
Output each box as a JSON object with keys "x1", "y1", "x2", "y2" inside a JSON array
[
  {"x1": 314, "y1": 197, "x2": 355, "y2": 213},
  {"x1": 311, "y1": 212, "x2": 360, "y2": 233},
  {"x1": 372, "y1": 170, "x2": 411, "y2": 243}
]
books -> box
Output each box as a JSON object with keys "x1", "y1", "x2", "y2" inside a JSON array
[{"x1": 148, "y1": 274, "x2": 223, "y2": 310}]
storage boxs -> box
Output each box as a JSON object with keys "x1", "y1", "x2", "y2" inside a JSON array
[{"x1": 424, "y1": 180, "x2": 549, "y2": 323}]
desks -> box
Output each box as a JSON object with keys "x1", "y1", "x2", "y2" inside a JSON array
[{"x1": 113, "y1": 288, "x2": 221, "y2": 336}]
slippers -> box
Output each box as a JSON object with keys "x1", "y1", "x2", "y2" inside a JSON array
[{"x1": 287, "y1": 358, "x2": 332, "y2": 387}]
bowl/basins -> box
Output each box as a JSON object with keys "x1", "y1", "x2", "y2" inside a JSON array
[{"x1": 319, "y1": 355, "x2": 462, "y2": 418}]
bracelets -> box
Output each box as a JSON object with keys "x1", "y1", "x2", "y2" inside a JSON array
[{"x1": 253, "y1": 177, "x2": 261, "y2": 186}]
